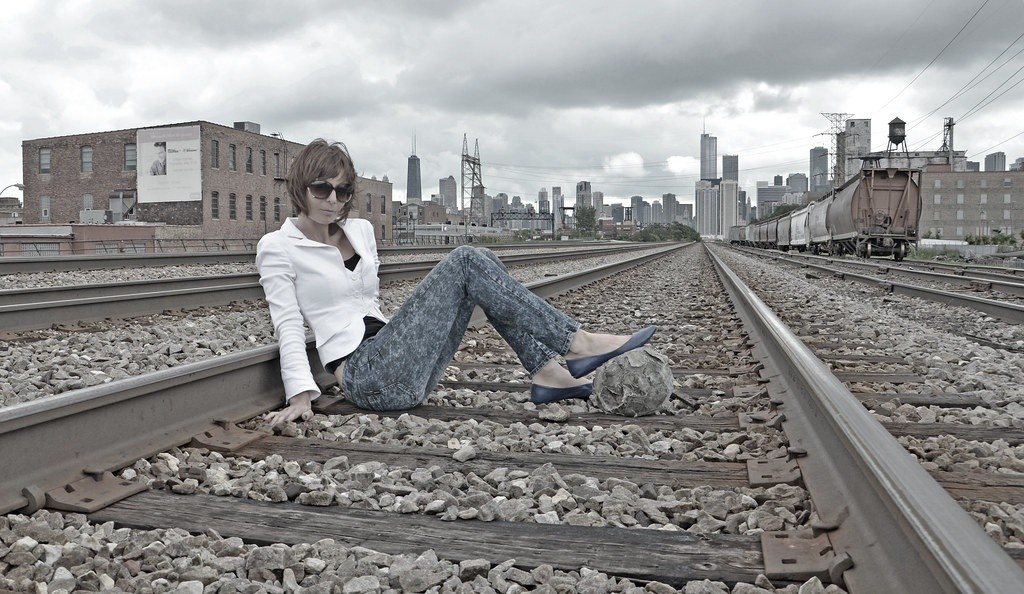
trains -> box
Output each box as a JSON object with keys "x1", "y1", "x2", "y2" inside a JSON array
[{"x1": 728, "y1": 167, "x2": 923, "y2": 261}]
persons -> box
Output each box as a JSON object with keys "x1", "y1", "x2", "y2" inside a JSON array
[
  {"x1": 149, "y1": 141, "x2": 167, "y2": 176},
  {"x1": 254, "y1": 139, "x2": 657, "y2": 428}
]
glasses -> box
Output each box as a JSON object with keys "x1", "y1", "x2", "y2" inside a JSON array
[{"x1": 307, "y1": 181, "x2": 356, "y2": 204}]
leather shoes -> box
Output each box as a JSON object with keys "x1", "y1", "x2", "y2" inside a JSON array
[
  {"x1": 531, "y1": 382, "x2": 595, "y2": 406},
  {"x1": 565, "y1": 324, "x2": 658, "y2": 380}
]
